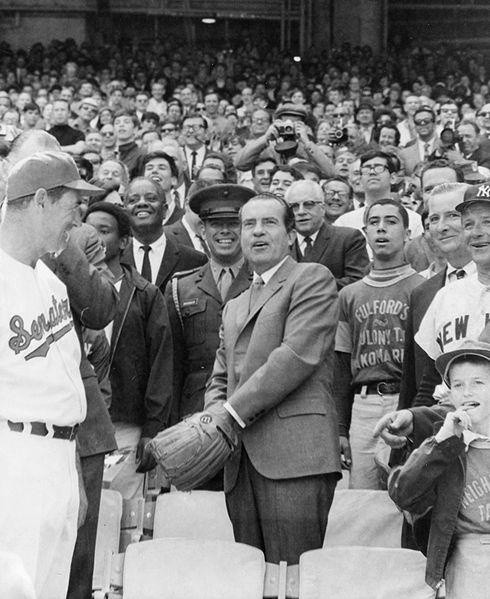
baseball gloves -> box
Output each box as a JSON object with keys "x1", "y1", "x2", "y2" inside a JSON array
[{"x1": 148, "y1": 402, "x2": 239, "y2": 494}]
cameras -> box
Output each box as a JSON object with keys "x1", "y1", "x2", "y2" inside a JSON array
[
  {"x1": 329, "y1": 128, "x2": 348, "y2": 142},
  {"x1": 279, "y1": 122, "x2": 295, "y2": 135}
]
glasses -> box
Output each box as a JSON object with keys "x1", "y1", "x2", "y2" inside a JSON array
[
  {"x1": 377, "y1": 119, "x2": 392, "y2": 124},
  {"x1": 196, "y1": 107, "x2": 206, "y2": 111},
  {"x1": 288, "y1": 199, "x2": 324, "y2": 212},
  {"x1": 415, "y1": 118, "x2": 433, "y2": 124},
  {"x1": 82, "y1": 157, "x2": 100, "y2": 165},
  {"x1": 332, "y1": 113, "x2": 351, "y2": 117},
  {"x1": 478, "y1": 112, "x2": 490, "y2": 117},
  {"x1": 361, "y1": 162, "x2": 391, "y2": 175},
  {"x1": 440, "y1": 109, "x2": 460, "y2": 113},
  {"x1": 83, "y1": 107, "x2": 98, "y2": 114},
  {"x1": 182, "y1": 124, "x2": 206, "y2": 131},
  {"x1": 323, "y1": 189, "x2": 351, "y2": 200}
]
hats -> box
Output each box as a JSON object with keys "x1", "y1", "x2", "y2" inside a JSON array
[
  {"x1": 188, "y1": 183, "x2": 262, "y2": 223},
  {"x1": 432, "y1": 338, "x2": 490, "y2": 392},
  {"x1": 453, "y1": 183, "x2": 490, "y2": 213},
  {"x1": 3, "y1": 148, "x2": 108, "y2": 208}
]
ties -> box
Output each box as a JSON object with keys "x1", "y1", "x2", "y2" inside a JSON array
[
  {"x1": 246, "y1": 279, "x2": 266, "y2": 320},
  {"x1": 172, "y1": 190, "x2": 182, "y2": 209},
  {"x1": 423, "y1": 142, "x2": 432, "y2": 163},
  {"x1": 137, "y1": 244, "x2": 155, "y2": 284},
  {"x1": 455, "y1": 269, "x2": 466, "y2": 281},
  {"x1": 190, "y1": 150, "x2": 199, "y2": 181},
  {"x1": 196, "y1": 233, "x2": 213, "y2": 262},
  {"x1": 359, "y1": 203, "x2": 364, "y2": 208},
  {"x1": 304, "y1": 236, "x2": 314, "y2": 261}
]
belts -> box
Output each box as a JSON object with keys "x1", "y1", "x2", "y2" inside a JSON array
[{"x1": 354, "y1": 378, "x2": 412, "y2": 398}]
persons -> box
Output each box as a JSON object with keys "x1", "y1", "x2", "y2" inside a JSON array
[
  {"x1": 386, "y1": 338, "x2": 489, "y2": 599},
  {"x1": 0, "y1": 32, "x2": 489, "y2": 499},
  {"x1": 202, "y1": 192, "x2": 342, "y2": 564},
  {"x1": 82, "y1": 200, "x2": 174, "y2": 557}
]
